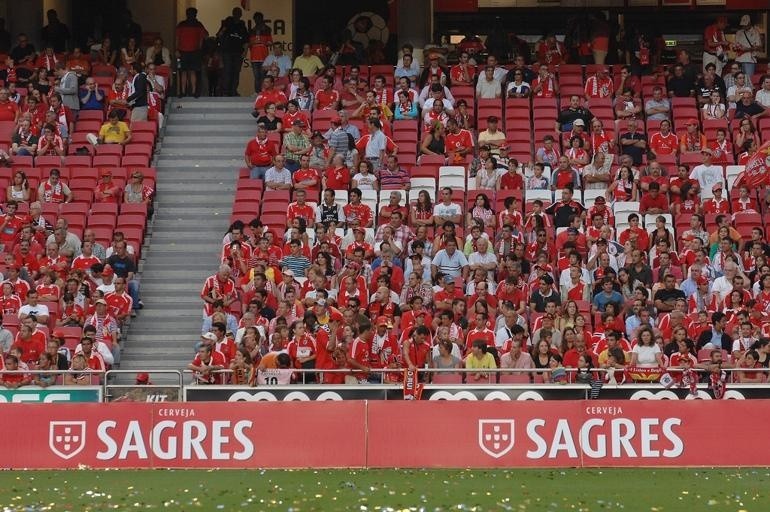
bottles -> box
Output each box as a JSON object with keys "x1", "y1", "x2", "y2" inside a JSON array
[
  {"x1": 177, "y1": 58, "x2": 182, "y2": 68},
  {"x1": 292, "y1": 308, "x2": 296, "y2": 320}
]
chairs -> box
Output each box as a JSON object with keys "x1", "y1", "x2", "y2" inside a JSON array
[
  {"x1": 228, "y1": 61, "x2": 769, "y2": 383},
  {"x1": 0, "y1": 32, "x2": 182, "y2": 382}
]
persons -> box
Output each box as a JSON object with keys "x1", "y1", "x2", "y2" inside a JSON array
[{"x1": 2, "y1": 1, "x2": 770, "y2": 391}]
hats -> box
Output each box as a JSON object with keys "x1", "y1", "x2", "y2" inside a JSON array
[
  {"x1": 5, "y1": 264, "x2": 17, "y2": 270},
  {"x1": 20, "y1": 214, "x2": 33, "y2": 225},
  {"x1": 102, "y1": 266, "x2": 113, "y2": 275},
  {"x1": 202, "y1": 51, "x2": 726, "y2": 361},
  {"x1": 101, "y1": 170, "x2": 112, "y2": 177},
  {"x1": 50, "y1": 328, "x2": 64, "y2": 338},
  {"x1": 138, "y1": 371, "x2": 150, "y2": 382},
  {"x1": 96, "y1": 298, "x2": 106, "y2": 306}
]
